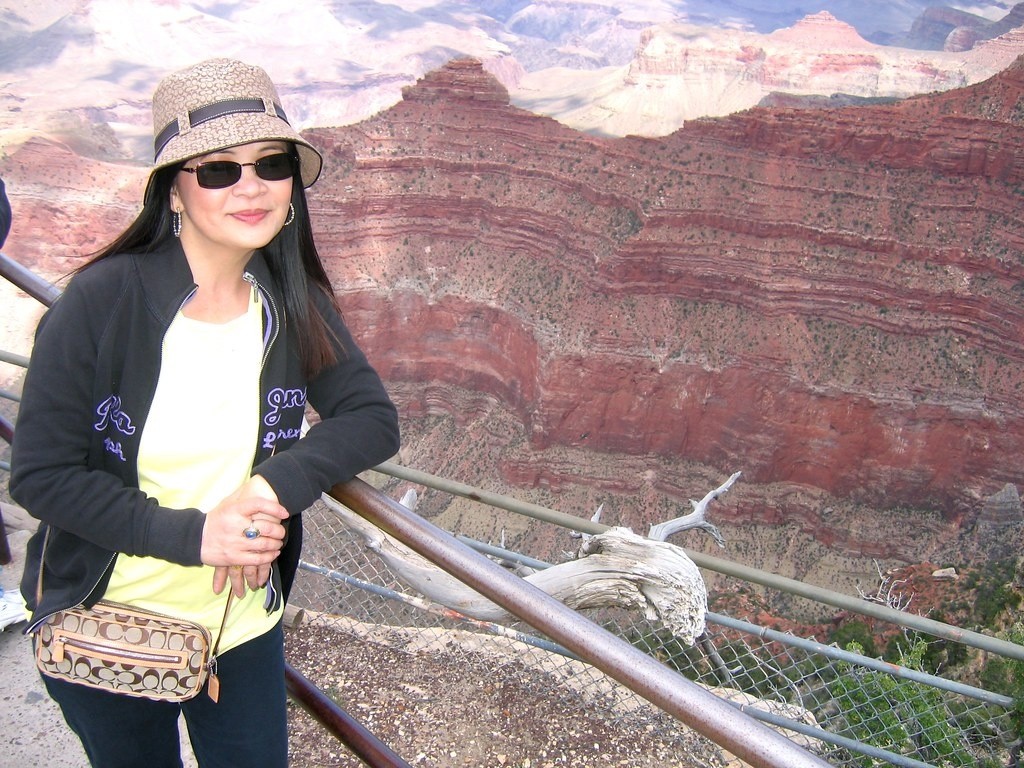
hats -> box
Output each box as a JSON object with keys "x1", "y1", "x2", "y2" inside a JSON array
[{"x1": 143, "y1": 57, "x2": 324, "y2": 207}]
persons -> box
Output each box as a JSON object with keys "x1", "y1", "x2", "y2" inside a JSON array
[{"x1": 8, "y1": 60, "x2": 400, "y2": 768}]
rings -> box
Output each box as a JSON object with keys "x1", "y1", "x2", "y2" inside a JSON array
[{"x1": 242, "y1": 520, "x2": 260, "y2": 540}]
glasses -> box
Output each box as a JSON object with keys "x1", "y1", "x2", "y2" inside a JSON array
[{"x1": 179, "y1": 152, "x2": 301, "y2": 189}]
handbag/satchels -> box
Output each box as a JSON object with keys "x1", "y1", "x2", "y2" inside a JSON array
[{"x1": 31, "y1": 597, "x2": 219, "y2": 703}]
